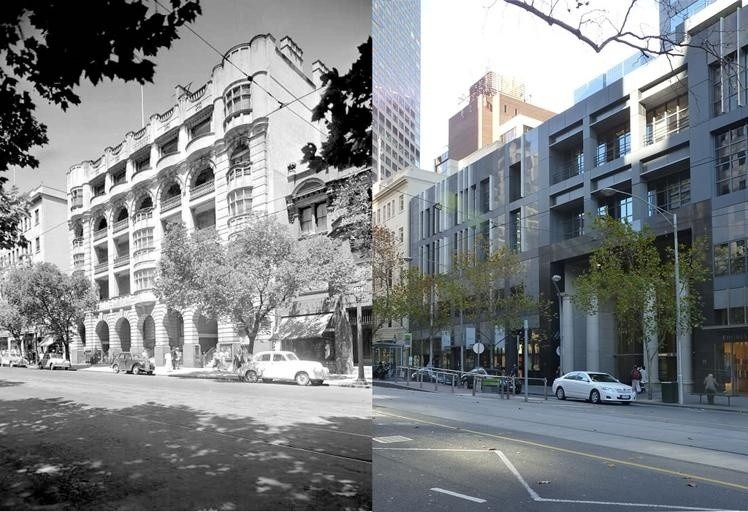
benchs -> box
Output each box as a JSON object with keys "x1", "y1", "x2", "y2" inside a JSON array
[{"x1": 698, "y1": 393, "x2": 739, "y2": 407}]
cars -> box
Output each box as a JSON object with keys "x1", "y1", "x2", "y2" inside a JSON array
[
  {"x1": 234, "y1": 350, "x2": 331, "y2": 389},
  {"x1": 549, "y1": 369, "x2": 638, "y2": 407},
  {"x1": 111, "y1": 350, "x2": 157, "y2": 379},
  {"x1": 0, "y1": 348, "x2": 72, "y2": 371},
  {"x1": 397, "y1": 364, "x2": 522, "y2": 399}
]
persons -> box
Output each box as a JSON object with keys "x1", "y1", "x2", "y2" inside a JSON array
[
  {"x1": 630, "y1": 365, "x2": 642, "y2": 394},
  {"x1": 509, "y1": 364, "x2": 523, "y2": 382},
  {"x1": 171, "y1": 346, "x2": 182, "y2": 370},
  {"x1": 637, "y1": 365, "x2": 648, "y2": 393},
  {"x1": 210, "y1": 346, "x2": 251, "y2": 383},
  {"x1": 702, "y1": 372, "x2": 720, "y2": 405}
]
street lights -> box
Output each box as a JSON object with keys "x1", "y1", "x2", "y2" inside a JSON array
[
  {"x1": 550, "y1": 271, "x2": 563, "y2": 379},
  {"x1": 403, "y1": 256, "x2": 464, "y2": 373},
  {"x1": 601, "y1": 186, "x2": 683, "y2": 405},
  {"x1": 351, "y1": 283, "x2": 369, "y2": 388}
]
